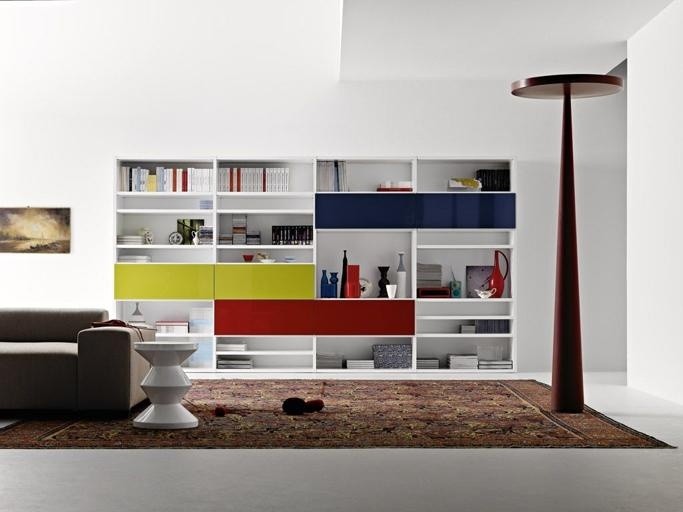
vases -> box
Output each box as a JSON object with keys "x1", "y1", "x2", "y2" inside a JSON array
[
  {"x1": 396, "y1": 252, "x2": 407, "y2": 298},
  {"x1": 378, "y1": 266, "x2": 390, "y2": 298},
  {"x1": 489, "y1": 250, "x2": 504, "y2": 298}
]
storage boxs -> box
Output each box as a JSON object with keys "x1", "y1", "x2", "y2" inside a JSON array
[
  {"x1": 155, "y1": 320, "x2": 189, "y2": 334},
  {"x1": 372, "y1": 344, "x2": 412, "y2": 369}
]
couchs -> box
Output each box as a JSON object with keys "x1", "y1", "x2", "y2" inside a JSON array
[{"x1": 0, "y1": 306, "x2": 157, "y2": 418}]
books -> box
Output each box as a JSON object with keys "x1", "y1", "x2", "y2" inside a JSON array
[
  {"x1": 120, "y1": 165, "x2": 289, "y2": 192},
  {"x1": 416, "y1": 354, "x2": 512, "y2": 369},
  {"x1": 118, "y1": 255, "x2": 152, "y2": 263},
  {"x1": 342, "y1": 359, "x2": 374, "y2": 369},
  {"x1": 216, "y1": 344, "x2": 253, "y2": 369},
  {"x1": 117, "y1": 234, "x2": 145, "y2": 244},
  {"x1": 272, "y1": 225, "x2": 313, "y2": 245},
  {"x1": 476, "y1": 168, "x2": 510, "y2": 192},
  {"x1": 318, "y1": 160, "x2": 349, "y2": 192}
]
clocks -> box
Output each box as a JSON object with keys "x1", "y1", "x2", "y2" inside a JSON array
[{"x1": 168, "y1": 231, "x2": 185, "y2": 245}]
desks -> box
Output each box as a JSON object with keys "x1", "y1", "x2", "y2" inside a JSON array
[{"x1": 132, "y1": 342, "x2": 199, "y2": 429}]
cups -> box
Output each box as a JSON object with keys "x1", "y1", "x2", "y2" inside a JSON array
[{"x1": 385, "y1": 284, "x2": 397, "y2": 297}]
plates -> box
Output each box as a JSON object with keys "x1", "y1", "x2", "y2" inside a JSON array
[
  {"x1": 284, "y1": 260, "x2": 297, "y2": 262},
  {"x1": 259, "y1": 259, "x2": 275, "y2": 263}
]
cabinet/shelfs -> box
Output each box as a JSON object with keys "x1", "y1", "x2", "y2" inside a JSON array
[{"x1": 112, "y1": 156, "x2": 519, "y2": 377}]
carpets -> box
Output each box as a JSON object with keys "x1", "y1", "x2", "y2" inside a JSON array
[{"x1": 0, "y1": 378, "x2": 679, "y2": 450}]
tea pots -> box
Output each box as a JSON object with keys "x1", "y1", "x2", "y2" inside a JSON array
[{"x1": 473, "y1": 287, "x2": 497, "y2": 298}]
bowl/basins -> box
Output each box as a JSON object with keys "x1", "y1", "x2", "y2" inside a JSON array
[{"x1": 242, "y1": 254, "x2": 254, "y2": 262}]
